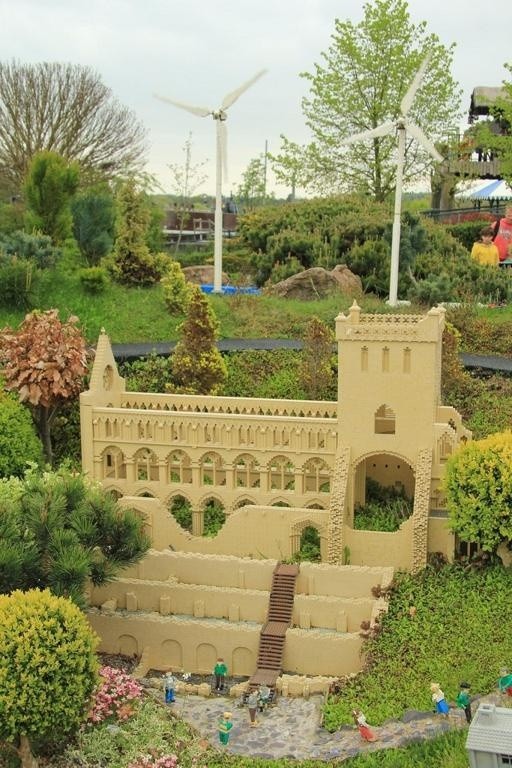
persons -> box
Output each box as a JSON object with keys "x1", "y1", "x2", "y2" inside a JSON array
[
  {"x1": 498, "y1": 666, "x2": 512, "y2": 696},
  {"x1": 162, "y1": 668, "x2": 178, "y2": 703},
  {"x1": 489, "y1": 208, "x2": 511, "y2": 262},
  {"x1": 257, "y1": 684, "x2": 270, "y2": 712},
  {"x1": 248, "y1": 689, "x2": 259, "y2": 725},
  {"x1": 211, "y1": 657, "x2": 228, "y2": 692},
  {"x1": 159, "y1": 655, "x2": 511, "y2": 749},
  {"x1": 457, "y1": 683, "x2": 474, "y2": 724},
  {"x1": 352, "y1": 709, "x2": 378, "y2": 742},
  {"x1": 217, "y1": 712, "x2": 233, "y2": 746},
  {"x1": 470, "y1": 222, "x2": 500, "y2": 270},
  {"x1": 429, "y1": 682, "x2": 450, "y2": 720}
]
